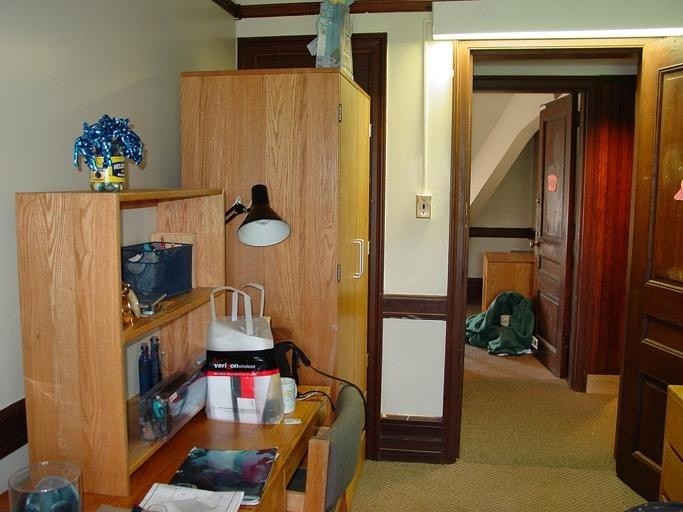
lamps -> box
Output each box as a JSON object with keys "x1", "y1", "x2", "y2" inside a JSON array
[{"x1": 224, "y1": 182, "x2": 293, "y2": 248}]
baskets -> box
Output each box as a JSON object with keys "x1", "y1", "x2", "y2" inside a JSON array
[{"x1": 121, "y1": 242, "x2": 193, "y2": 301}]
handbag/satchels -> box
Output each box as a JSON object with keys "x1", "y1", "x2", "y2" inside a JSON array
[{"x1": 207, "y1": 284, "x2": 284, "y2": 425}]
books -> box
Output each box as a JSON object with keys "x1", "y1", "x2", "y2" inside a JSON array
[{"x1": 166, "y1": 445, "x2": 279, "y2": 505}]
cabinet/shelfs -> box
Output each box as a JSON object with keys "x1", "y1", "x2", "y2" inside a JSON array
[
  {"x1": 482, "y1": 250, "x2": 536, "y2": 312},
  {"x1": 177, "y1": 71, "x2": 371, "y2": 407},
  {"x1": 14, "y1": 185, "x2": 228, "y2": 501}
]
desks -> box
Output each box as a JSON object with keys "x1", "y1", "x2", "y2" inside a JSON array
[{"x1": 1, "y1": 382, "x2": 331, "y2": 512}]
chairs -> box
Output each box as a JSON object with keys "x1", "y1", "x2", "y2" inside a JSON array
[
  {"x1": 659, "y1": 384, "x2": 683, "y2": 501},
  {"x1": 276, "y1": 381, "x2": 370, "y2": 512}
]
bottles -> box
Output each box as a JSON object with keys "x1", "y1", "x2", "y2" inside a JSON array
[{"x1": 139, "y1": 336, "x2": 162, "y2": 399}]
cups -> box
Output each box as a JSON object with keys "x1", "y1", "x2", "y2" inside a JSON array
[{"x1": 279, "y1": 377, "x2": 298, "y2": 414}]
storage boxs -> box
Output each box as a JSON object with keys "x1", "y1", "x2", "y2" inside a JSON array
[{"x1": 120, "y1": 237, "x2": 197, "y2": 304}]
